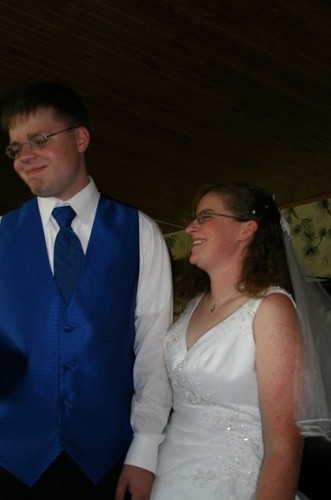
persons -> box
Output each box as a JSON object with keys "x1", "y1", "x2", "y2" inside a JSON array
[
  {"x1": 0, "y1": 88, "x2": 175, "y2": 500},
  {"x1": 159, "y1": 185, "x2": 331, "y2": 500}
]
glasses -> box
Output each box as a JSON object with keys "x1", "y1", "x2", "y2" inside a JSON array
[
  {"x1": 192, "y1": 209, "x2": 249, "y2": 225},
  {"x1": 6, "y1": 125, "x2": 81, "y2": 159}
]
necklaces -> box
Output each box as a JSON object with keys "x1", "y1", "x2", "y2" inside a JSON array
[{"x1": 209, "y1": 291, "x2": 249, "y2": 313}]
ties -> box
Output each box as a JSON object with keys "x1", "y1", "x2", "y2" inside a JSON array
[{"x1": 52, "y1": 205, "x2": 84, "y2": 307}]
